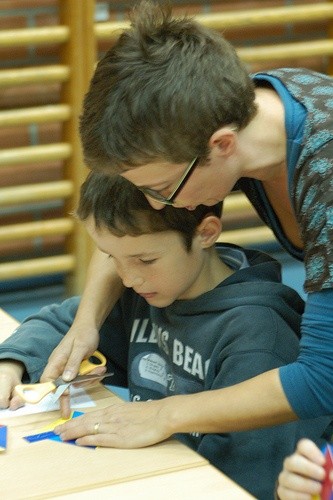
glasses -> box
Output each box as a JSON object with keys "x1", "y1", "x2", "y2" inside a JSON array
[{"x1": 135, "y1": 156, "x2": 200, "y2": 207}]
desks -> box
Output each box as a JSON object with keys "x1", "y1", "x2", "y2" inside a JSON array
[{"x1": 0, "y1": 308, "x2": 258, "y2": 500}]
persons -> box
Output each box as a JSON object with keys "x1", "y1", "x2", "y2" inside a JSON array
[
  {"x1": 38, "y1": 0, "x2": 333, "y2": 447},
  {"x1": 0, "y1": 168, "x2": 332, "y2": 500},
  {"x1": 275, "y1": 437, "x2": 333, "y2": 499}
]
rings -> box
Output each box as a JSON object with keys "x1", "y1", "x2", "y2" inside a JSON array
[{"x1": 94, "y1": 421, "x2": 100, "y2": 433}]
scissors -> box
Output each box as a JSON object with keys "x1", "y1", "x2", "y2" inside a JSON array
[{"x1": 14, "y1": 352, "x2": 115, "y2": 408}]
